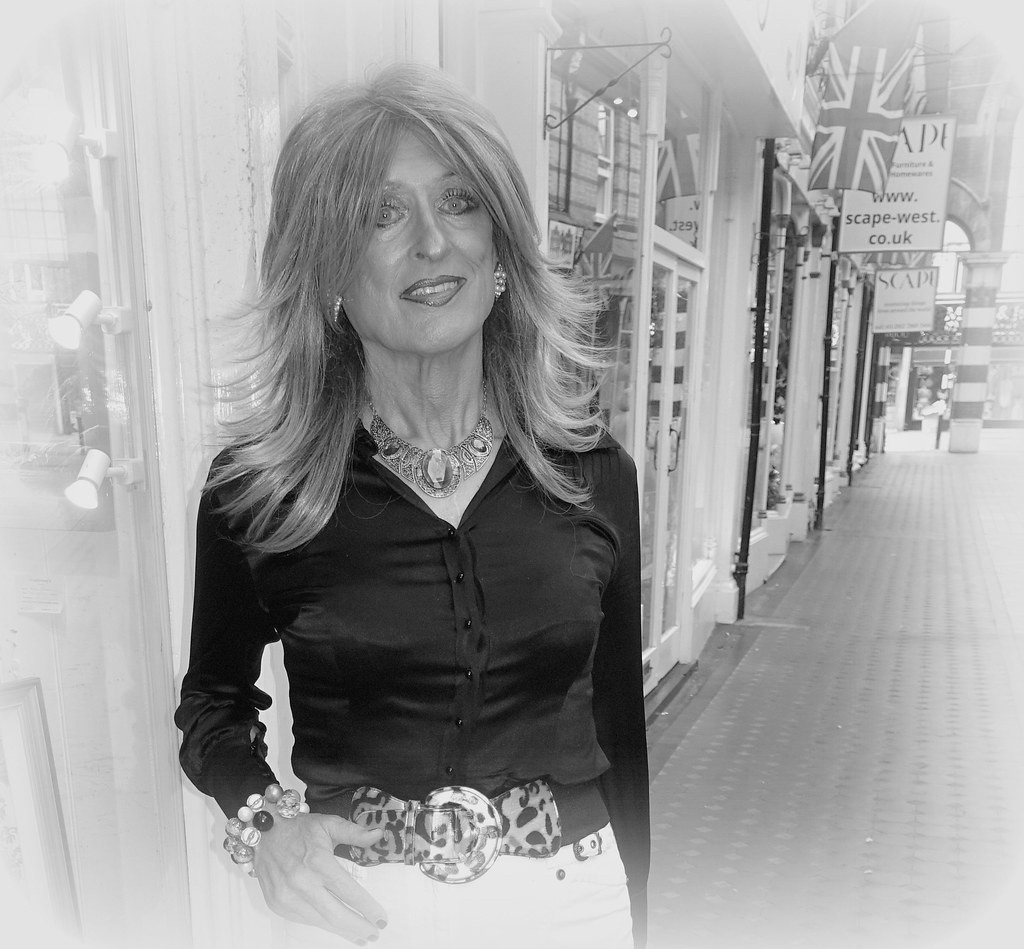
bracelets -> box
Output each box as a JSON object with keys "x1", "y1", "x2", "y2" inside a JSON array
[{"x1": 220, "y1": 785, "x2": 304, "y2": 875}]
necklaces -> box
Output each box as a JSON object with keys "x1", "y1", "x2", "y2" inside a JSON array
[{"x1": 359, "y1": 359, "x2": 496, "y2": 501}]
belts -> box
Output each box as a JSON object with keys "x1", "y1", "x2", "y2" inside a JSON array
[{"x1": 304, "y1": 778, "x2": 612, "y2": 884}]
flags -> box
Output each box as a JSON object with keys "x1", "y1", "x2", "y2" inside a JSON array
[{"x1": 807, "y1": 33, "x2": 918, "y2": 200}]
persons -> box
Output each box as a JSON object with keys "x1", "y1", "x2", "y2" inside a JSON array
[{"x1": 176, "y1": 71, "x2": 652, "y2": 949}]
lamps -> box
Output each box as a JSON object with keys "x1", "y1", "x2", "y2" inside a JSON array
[
  {"x1": 66, "y1": 449, "x2": 121, "y2": 511},
  {"x1": 48, "y1": 291, "x2": 114, "y2": 351}
]
